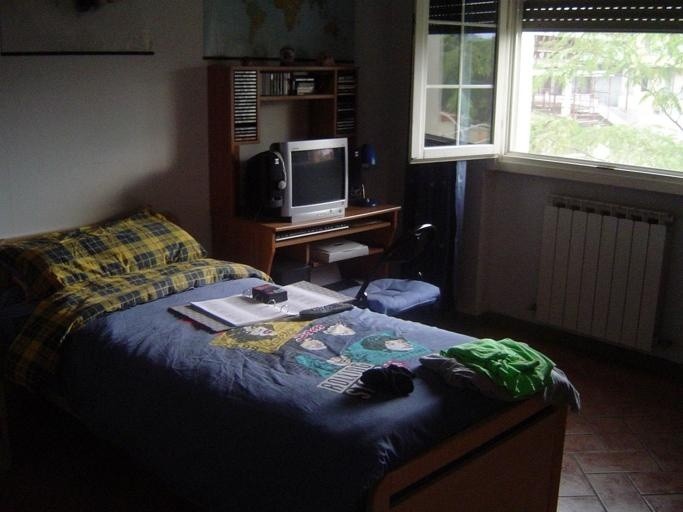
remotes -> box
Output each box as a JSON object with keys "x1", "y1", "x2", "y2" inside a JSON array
[{"x1": 298, "y1": 303, "x2": 354, "y2": 319}]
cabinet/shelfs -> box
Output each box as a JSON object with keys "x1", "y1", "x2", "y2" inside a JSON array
[{"x1": 207, "y1": 59, "x2": 405, "y2": 281}]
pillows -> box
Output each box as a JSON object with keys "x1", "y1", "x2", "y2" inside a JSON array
[{"x1": 1, "y1": 207, "x2": 208, "y2": 302}]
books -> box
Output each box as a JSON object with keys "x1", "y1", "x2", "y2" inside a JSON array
[
  {"x1": 233, "y1": 71, "x2": 258, "y2": 142},
  {"x1": 258, "y1": 71, "x2": 315, "y2": 96},
  {"x1": 166, "y1": 304, "x2": 234, "y2": 333},
  {"x1": 312, "y1": 239, "x2": 369, "y2": 264},
  {"x1": 188, "y1": 280, "x2": 354, "y2": 328},
  {"x1": 335, "y1": 74, "x2": 355, "y2": 134}
]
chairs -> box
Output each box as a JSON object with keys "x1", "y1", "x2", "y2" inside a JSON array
[{"x1": 337, "y1": 219, "x2": 442, "y2": 324}]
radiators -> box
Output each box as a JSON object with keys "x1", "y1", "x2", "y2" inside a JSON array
[{"x1": 534, "y1": 201, "x2": 673, "y2": 355}]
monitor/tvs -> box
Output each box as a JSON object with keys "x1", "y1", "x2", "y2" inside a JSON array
[{"x1": 269, "y1": 137, "x2": 351, "y2": 224}]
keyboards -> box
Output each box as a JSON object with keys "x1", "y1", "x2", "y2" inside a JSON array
[{"x1": 274, "y1": 222, "x2": 350, "y2": 242}]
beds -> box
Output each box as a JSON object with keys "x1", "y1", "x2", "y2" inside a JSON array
[{"x1": 2, "y1": 204, "x2": 572, "y2": 510}]
